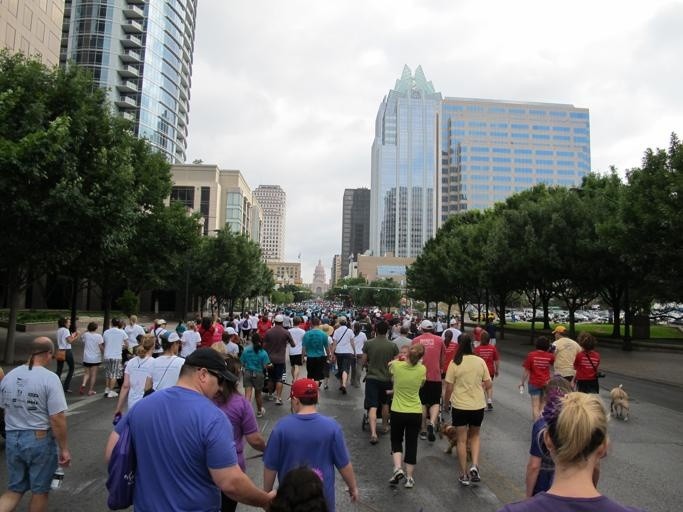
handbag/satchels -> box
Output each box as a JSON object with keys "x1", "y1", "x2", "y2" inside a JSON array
[
  {"x1": 106, "y1": 423, "x2": 134, "y2": 510},
  {"x1": 55, "y1": 351, "x2": 65, "y2": 360}
]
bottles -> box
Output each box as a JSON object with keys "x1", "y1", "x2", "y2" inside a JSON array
[
  {"x1": 113, "y1": 412, "x2": 121, "y2": 425},
  {"x1": 519, "y1": 385, "x2": 524, "y2": 394},
  {"x1": 49, "y1": 462, "x2": 64, "y2": 490},
  {"x1": 331, "y1": 362, "x2": 335, "y2": 373}
]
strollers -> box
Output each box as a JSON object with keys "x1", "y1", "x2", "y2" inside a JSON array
[
  {"x1": 361, "y1": 365, "x2": 392, "y2": 430},
  {"x1": 436, "y1": 396, "x2": 445, "y2": 439}
]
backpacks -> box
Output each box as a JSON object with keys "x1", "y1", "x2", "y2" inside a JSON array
[
  {"x1": 243, "y1": 320, "x2": 248, "y2": 328},
  {"x1": 153, "y1": 330, "x2": 161, "y2": 349}
]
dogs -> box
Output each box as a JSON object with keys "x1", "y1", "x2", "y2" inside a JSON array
[
  {"x1": 439, "y1": 422, "x2": 472, "y2": 462},
  {"x1": 609, "y1": 384, "x2": 631, "y2": 422}
]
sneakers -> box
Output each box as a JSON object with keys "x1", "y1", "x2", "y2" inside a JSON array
[
  {"x1": 80, "y1": 385, "x2": 85, "y2": 394},
  {"x1": 419, "y1": 425, "x2": 436, "y2": 442},
  {"x1": 459, "y1": 465, "x2": 481, "y2": 486},
  {"x1": 382, "y1": 425, "x2": 391, "y2": 434},
  {"x1": 369, "y1": 433, "x2": 378, "y2": 444},
  {"x1": 390, "y1": 469, "x2": 415, "y2": 488},
  {"x1": 268, "y1": 395, "x2": 273, "y2": 400},
  {"x1": 88, "y1": 391, "x2": 96, "y2": 395},
  {"x1": 104, "y1": 389, "x2": 119, "y2": 398},
  {"x1": 339, "y1": 386, "x2": 347, "y2": 394},
  {"x1": 487, "y1": 401, "x2": 494, "y2": 410},
  {"x1": 275, "y1": 399, "x2": 283, "y2": 405},
  {"x1": 64, "y1": 389, "x2": 72, "y2": 393},
  {"x1": 257, "y1": 408, "x2": 265, "y2": 417}
]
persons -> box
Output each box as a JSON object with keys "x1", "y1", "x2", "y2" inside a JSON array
[
  {"x1": 503, "y1": 392, "x2": 638, "y2": 512},
  {"x1": 518, "y1": 326, "x2": 601, "y2": 497},
  {"x1": 1, "y1": 296, "x2": 503, "y2": 511}
]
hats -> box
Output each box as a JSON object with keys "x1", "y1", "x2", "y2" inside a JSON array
[
  {"x1": 450, "y1": 319, "x2": 456, "y2": 324},
  {"x1": 186, "y1": 348, "x2": 239, "y2": 382},
  {"x1": 157, "y1": 319, "x2": 166, "y2": 325},
  {"x1": 552, "y1": 326, "x2": 566, "y2": 334},
  {"x1": 224, "y1": 327, "x2": 238, "y2": 336},
  {"x1": 162, "y1": 331, "x2": 181, "y2": 342},
  {"x1": 275, "y1": 315, "x2": 283, "y2": 323},
  {"x1": 319, "y1": 324, "x2": 334, "y2": 335},
  {"x1": 421, "y1": 320, "x2": 434, "y2": 330},
  {"x1": 290, "y1": 379, "x2": 319, "y2": 398}
]
